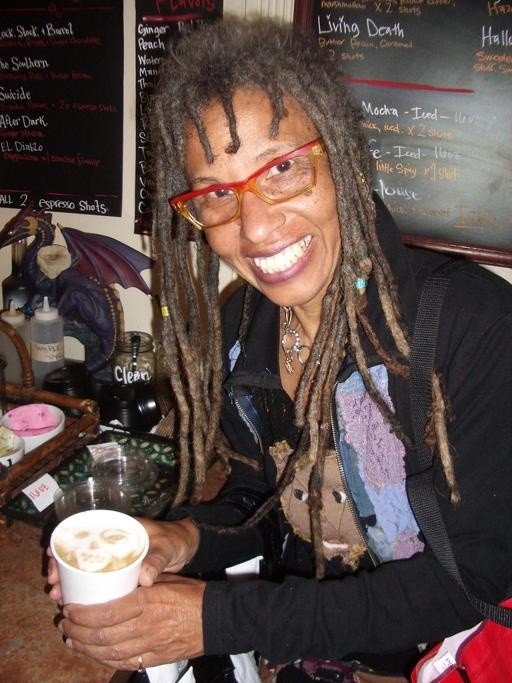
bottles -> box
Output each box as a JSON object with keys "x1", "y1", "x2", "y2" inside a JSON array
[
  {"x1": 30, "y1": 297, "x2": 64, "y2": 389},
  {"x1": 115, "y1": 332, "x2": 156, "y2": 392},
  {"x1": 1, "y1": 301, "x2": 31, "y2": 385}
]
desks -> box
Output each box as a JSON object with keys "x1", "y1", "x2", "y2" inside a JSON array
[{"x1": 0, "y1": 513, "x2": 117, "y2": 683}]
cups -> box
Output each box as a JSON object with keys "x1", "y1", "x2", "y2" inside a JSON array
[
  {"x1": 50, "y1": 446, "x2": 158, "y2": 605},
  {"x1": 1, "y1": 403, "x2": 64, "y2": 453},
  {"x1": 1, "y1": 428, "x2": 24, "y2": 469}
]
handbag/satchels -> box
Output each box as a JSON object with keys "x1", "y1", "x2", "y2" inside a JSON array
[{"x1": 410, "y1": 597, "x2": 512, "y2": 683}]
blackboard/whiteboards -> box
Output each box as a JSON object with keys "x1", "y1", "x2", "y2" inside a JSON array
[{"x1": 294, "y1": 0, "x2": 512, "y2": 268}]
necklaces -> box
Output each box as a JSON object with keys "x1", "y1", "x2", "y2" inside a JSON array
[{"x1": 279, "y1": 304, "x2": 321, "y2": 376}]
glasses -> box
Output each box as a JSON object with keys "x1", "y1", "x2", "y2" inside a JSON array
[{"x1": 169, "y1": 136, "x2": 326, "y2": 231}]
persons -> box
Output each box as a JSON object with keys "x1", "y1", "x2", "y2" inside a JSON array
[{"x1": 44, "y1": 18, "x2": 511, "y2": 683}]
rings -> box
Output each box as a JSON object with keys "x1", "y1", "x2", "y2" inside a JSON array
[{"x1": 139, "y1": 657, "x2": 144, "y2": 676}]
